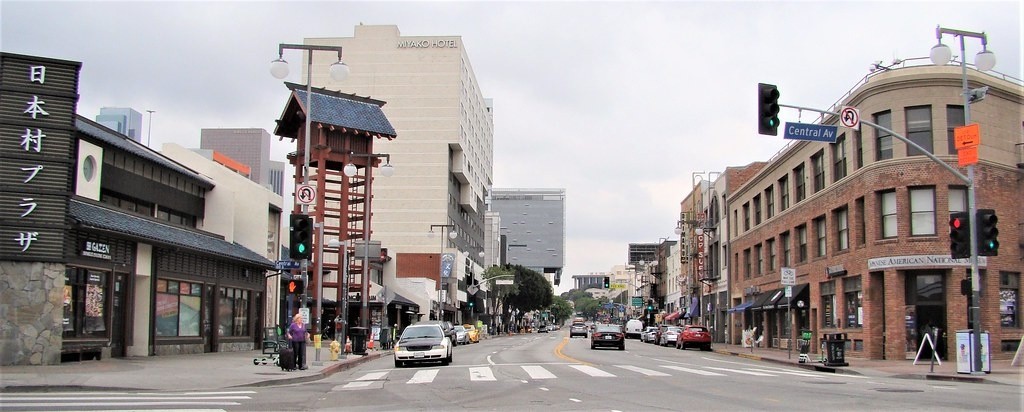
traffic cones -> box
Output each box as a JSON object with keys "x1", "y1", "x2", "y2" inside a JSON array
[
  {"x1": 344, "y1": 336, "x2": 352, "y2": 353},
  {"x1": 367, "y1": 332, "x2": 376, "y2": 348}
]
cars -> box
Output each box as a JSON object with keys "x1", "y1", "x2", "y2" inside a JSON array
[
  {"x1": 641, "y1": 324, "x2": 712, "y2": 350},
  {"x1": 538, "y1": 323, "x2": 560, "y2": 333},
  {"x1": 569, "y1": 316, "x2": 589, "y2": 338},
  {"x1": 393, "y1": 320, "x2": 481, "y2": 366},
  {"x1": 591, "y1": 324, "x2": 626, "y2": 350}
]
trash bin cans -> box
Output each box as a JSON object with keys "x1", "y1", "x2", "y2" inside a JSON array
[
  {"x1": 819, "y1": 332, "x2": 849, "y2": 365},
  {"x1": 349, "y1": 327, "x2": 370, "y2": 355}
]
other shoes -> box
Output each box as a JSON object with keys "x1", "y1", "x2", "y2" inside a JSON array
[{"x1": 299, "y1": 366, "x2": 306, "y2": 370}]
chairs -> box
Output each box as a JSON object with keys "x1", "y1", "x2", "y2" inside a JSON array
[{"x1": 263, "y1": 326, "x2": 286, "y2": 359}]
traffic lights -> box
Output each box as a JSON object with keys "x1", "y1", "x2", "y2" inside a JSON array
[
  {"x1": 948, "y1": 211, "x2": 971, "y2": 259},
  {"x1": 977, "y1": 208, "x2": 999, "y2": 256},
  {"x1": 604, "y1": 277, "x2": 610, "y2": 289},
  {"x1": 469, "y1": 294, "x2": 476, "y2": 307},
  {"x1": 647, "y1": 299, "x2": 653, "y2": 310},
  {"x1": 288, "y1": 279, "x2": 305, "y2": 293},
  {"x1": 290, "y1": 214, "x2": 313, "y2": 260},
  {"x1": 757, "y1": 82, "x2": 780, "y2": 136}
]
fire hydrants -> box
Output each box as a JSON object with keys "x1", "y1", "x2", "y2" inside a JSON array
[{"x1": 328, "y1": 338, "x2": 341, "y2": 361}]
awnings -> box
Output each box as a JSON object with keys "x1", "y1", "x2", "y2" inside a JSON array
[
  {"x1": 726, "y1": 282, "x2": 809, "y2": 313},
  {"x1": 665, "y1": 302, "x2": 699, "y2": 320}
]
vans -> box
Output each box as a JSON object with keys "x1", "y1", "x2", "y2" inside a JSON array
[{"x1": 624, "y1": 319, "x2": 643, "y2": 339}]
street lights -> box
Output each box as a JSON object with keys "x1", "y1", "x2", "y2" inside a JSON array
[
  {"x1": 463, "y1": 244, "x2": 485, "y2": 320},
  {"x1": 638, "y1": 255, "x2": 658, "y2": 325},
  {"x1": 673, "y1": 220, "x2": 704, "y2": 324},
  {"x1": 928, "y1": 23, "x2": 997, "y2": 376},
  {"x1": 342, "y1": 150, "x2": 395, "y2": 329},
  {"x1": 427, "y1": 224, "x2": 458, "y2": 321},
  {"x1": 268, "y1": 42, "x2": 351, "y2": 309}
]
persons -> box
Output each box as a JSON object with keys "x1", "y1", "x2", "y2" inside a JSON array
[
  {"x1": 287, "y1": 314, "x2": 310, "y2": 370},
  {"x1": 334, "y1": 314, "x2": 346, "y2": 343}
]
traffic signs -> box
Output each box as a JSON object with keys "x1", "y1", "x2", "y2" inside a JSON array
[{"x1": 954, "y1": 123, "x2": 980, "y2": 148}]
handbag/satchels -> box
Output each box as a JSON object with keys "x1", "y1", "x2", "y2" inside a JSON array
[{"x1": 305, "y1": 331, "x2": 310, "y2": 344}]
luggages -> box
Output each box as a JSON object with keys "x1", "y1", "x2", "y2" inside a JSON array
[{"x1": 279, "y1": 339, "x2": 295, "y2": 371}]
